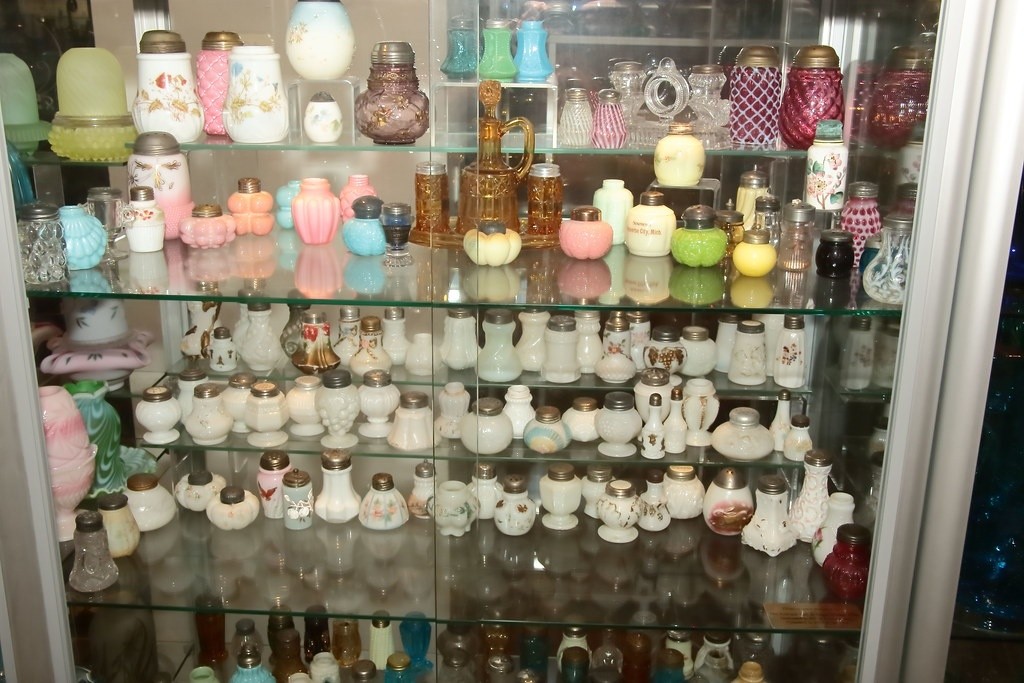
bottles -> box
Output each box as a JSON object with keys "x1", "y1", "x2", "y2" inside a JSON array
[{"x1": 0, "y1": 0, "x2": 928, "y2": 683}]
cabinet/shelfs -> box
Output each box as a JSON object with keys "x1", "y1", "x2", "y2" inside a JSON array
[{"x1": 0, "y1": 0, "x2": 1024, "y2": 683}]
